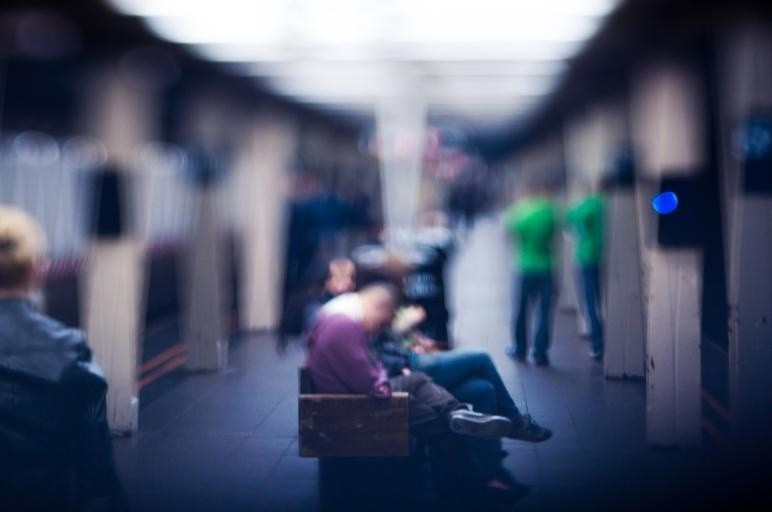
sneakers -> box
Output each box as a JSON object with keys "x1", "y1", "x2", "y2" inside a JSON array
[
  {"x1": 505, "y1": 412, "x2": 551, "y2": 442},
  {"x1": 448, "y1": 409, "x2": 513, "y2": 436},
  {"x1": 590, "y1": 348, "x2": 604, "y2": 360},
  {"x1": 505, "y1": 349, "x2": 549, "y2": 365}
]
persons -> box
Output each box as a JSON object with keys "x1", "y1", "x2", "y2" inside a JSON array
[
  {"x1": 297, "y1": 174, "x2": 608, "y2": 501},
  {"x1": 0, "y1": 205, "x2": 108, "y2": 511}
]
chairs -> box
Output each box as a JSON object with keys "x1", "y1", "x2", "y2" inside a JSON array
[{"x1": 294, "y1": 359, "x2": 430, "y2": 482}]
